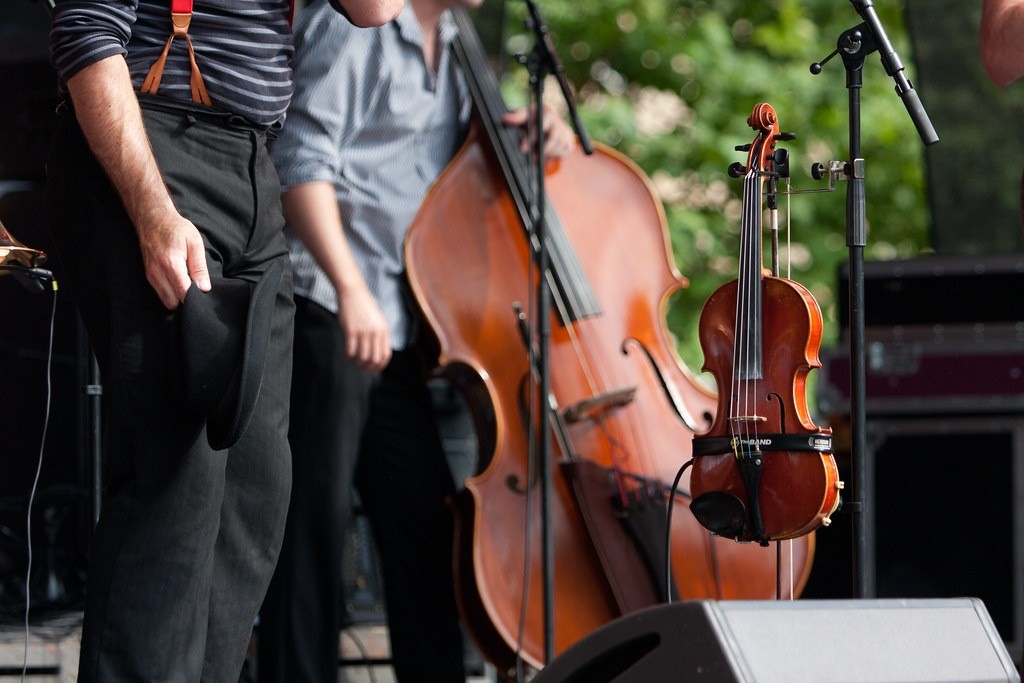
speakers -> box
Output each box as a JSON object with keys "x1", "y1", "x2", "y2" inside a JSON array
[{"x1": 528, "y1": 597, "x2": 1022, "y2": 683}]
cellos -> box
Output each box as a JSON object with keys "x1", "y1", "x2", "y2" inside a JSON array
[{"x1": 402, "y1": 4, "x2": 820, "y2": 678}]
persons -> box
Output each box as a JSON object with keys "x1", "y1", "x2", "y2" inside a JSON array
[
  {"x1": 268, "y1": 0, "x2": 575, "y2": 683},
  {"x1": 39, "y1": 0, "x2": 408, "y2": 683}
]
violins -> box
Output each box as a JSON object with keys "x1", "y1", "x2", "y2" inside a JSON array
[{"x1": 689, "y1": 102, "x2": 847, "y2": 549}]
